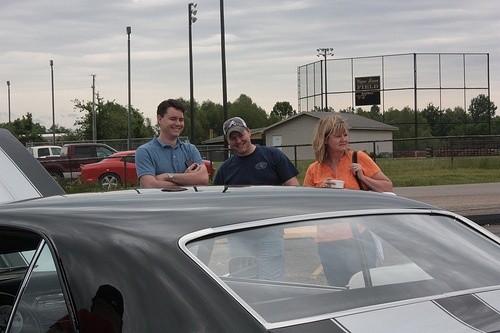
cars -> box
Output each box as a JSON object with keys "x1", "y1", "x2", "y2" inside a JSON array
[
  {"x1": 0, "y1": 127, "x2": 500, "y2": 333},
  {"x1": 77, "y1": 148, "x2": 216, "y2": 194},
  {"x1": 27, "y1": 145, "x2": 62, "y2": 159}
]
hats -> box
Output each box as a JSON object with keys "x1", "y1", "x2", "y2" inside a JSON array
[{"x1": 223, "y1": 117, "x2": 248, "y2": 136}]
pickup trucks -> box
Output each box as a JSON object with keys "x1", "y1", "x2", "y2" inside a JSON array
[{"x1": 34, "y1": 141, "x2": 121, "y2": 183}]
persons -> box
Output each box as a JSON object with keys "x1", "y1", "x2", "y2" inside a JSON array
[
  {"x1": 302, "y1": 115, "x2": 392, "y2": 286},
  {"x1": 135, "y1": 99, "x2": 208, "y2": 189},
  {"x1": 212, "y1": 117, "x2": 300, "y2": 282}
]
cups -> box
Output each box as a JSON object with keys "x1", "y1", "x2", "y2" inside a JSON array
[{"x1": 331, "y1": 180, "x2": 345, "y2": 188}]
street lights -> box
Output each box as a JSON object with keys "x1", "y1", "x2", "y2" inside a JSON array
[
  {"x1": 6, "y1": 80, "x2": 11, "y2": 122},
  {"x1": 126, "y1": 26, "x2": 133, "y2": 152},
  {"x1": 49, "y1": 59, "x2": 56, "y2": 145}
]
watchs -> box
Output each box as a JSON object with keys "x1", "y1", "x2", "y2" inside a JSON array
[{"x1": 168, "y1": 173, "x2": 175, "y2": 182}]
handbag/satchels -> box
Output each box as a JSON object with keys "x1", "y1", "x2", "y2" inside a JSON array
[{"x1": 361, "y1": 229, "x2": 384, "y2": 269}]
